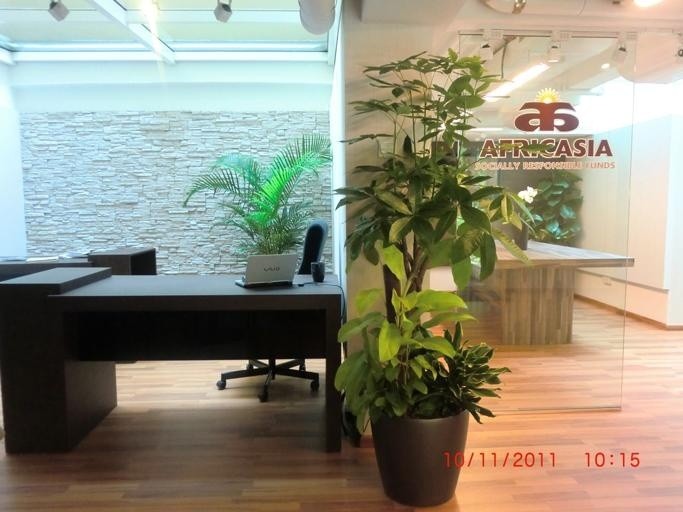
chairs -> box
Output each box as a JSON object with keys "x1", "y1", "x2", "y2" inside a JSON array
[{"x1": 216, "y1": 222, "x2": 329, "y2": 406}]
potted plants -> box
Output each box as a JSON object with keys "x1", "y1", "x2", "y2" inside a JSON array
[{"x1": 331, "y1": 45, "x2": 535, "y2": 507}]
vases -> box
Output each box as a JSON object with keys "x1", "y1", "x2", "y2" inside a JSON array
[{"x1": 512, "y1": 223, "x2": 529, "y2": 250}]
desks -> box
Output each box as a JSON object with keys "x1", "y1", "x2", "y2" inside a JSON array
[
  {"x1": 0, "y1": 247, "x2": 345, "y2": 458},
  {"x1": 473, "y1": 241, "x2": 636, "y2": 346}
]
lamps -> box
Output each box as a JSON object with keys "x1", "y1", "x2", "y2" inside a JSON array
[
  {"x1": 479, "y1": 35, "x2": 632, "y2": 64},
  {"x1": 213, "y1": 0, "x2": 234, "y2": 22},
  {"x1": 48, "y1": 0, "x2": 69, "y2": 21}
]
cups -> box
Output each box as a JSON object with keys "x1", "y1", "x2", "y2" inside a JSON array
[
  {"x1": 515, "y1": 224, "x2": 527, "y2": 250},
  {"x1": 311, "y1": 262, "x2": 325, "y2": 282}
]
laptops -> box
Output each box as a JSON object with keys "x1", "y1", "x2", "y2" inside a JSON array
[{"x1": 235, "y1": 255, "x2": 297, "y2": 288}]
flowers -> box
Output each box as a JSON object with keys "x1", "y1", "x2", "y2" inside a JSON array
[{"x1": 517, "y1": 185, "x2": 537, "y2": 222}]
